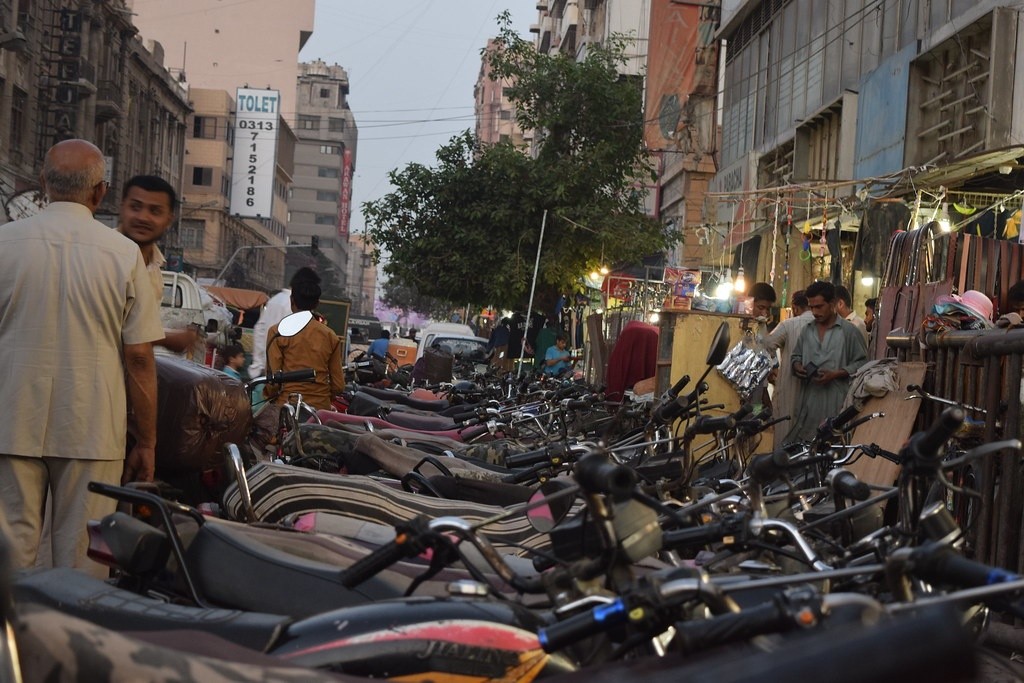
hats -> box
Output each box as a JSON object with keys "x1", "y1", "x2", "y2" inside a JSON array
[{"x1": 922, "y1": 289, "x2": 995, "y2": 331}]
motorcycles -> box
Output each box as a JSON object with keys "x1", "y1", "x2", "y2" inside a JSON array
[{"x1": 1, "y1": 290, "x2": 1024, "y2": 683}]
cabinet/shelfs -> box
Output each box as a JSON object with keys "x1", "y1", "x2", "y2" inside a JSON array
[{"x1": 654, "y1": 312, "x2": 759, "y2": 463}]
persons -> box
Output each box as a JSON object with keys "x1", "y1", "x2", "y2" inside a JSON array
[
  {"x1": 246, "y1": 268, "x2": 321, "y2": 380},
  {"x1": 544, "y1": 334, "x2": 579, "y2": 375},
  {"x1": 403, "y1": 328, "x2": 419, "y2": 343},
  {"x1": 863, "y1": 298, "x2": 877, "y2": 332},
  {"x1": 222, "y1": 345, "x2": 245, "y2": 380},
  {"x1": 0, "y1": 140, "x2": 165, "y2": 579},
  {"x1": 748, "y1": 282, "x2": 815, "y2": 445},
  {"x1": 263, "y1": 279, "x2": 346, "y2": 422},
  {"x1": 112, "y1": 173, "x2": 196, "y2": 353},
  {"x1": 789, "y1": 281, "x2": 868, "y2": 444},
  {"x1": 835, "y1": 285, "x2": 867, "y2": 341},
  {"x1": 368, "y1": 330, "x2": 390, "y2": 374}
]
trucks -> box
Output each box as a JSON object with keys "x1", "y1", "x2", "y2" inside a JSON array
[{"x1": 152, "y1": 269, "x2": 220, "y2": 366}]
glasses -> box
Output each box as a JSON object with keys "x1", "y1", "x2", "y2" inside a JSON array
[{"x1": 93, "y1": 180, "x2": 110, "y2": 192}]
